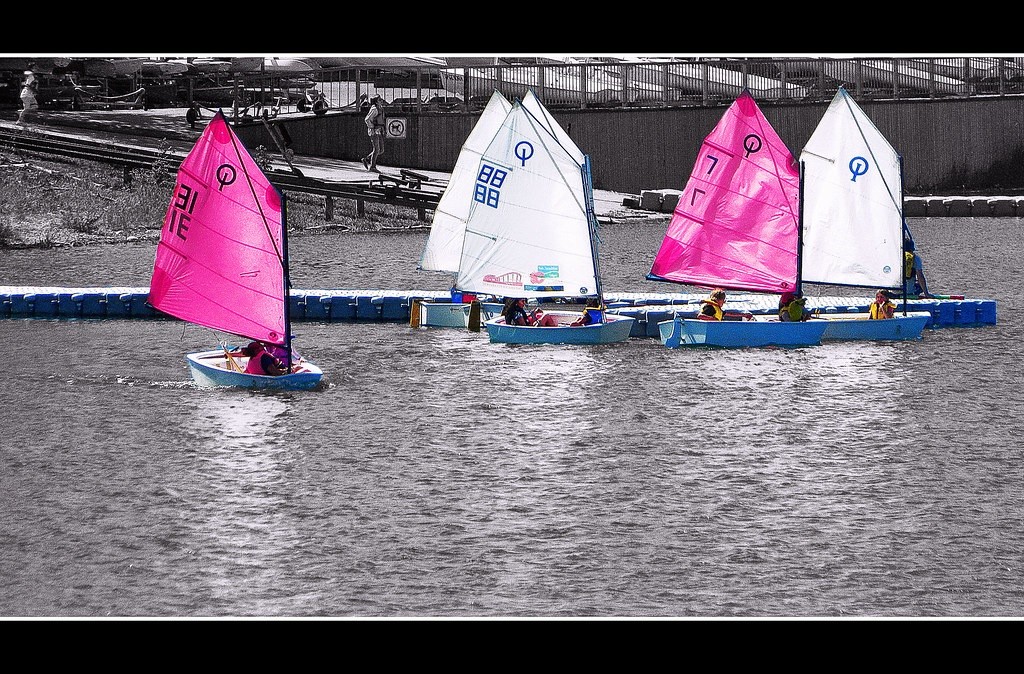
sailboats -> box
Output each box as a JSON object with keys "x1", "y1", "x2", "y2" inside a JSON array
[
  {"x1": 143, "y1": 106, "x2": 322, "y2": 391},
  {"x1": 410, "y1": 87, "x2": 636, "y2": 345},
  {"x1": 753, "y1": 81, "x2": 931, "y2": 341},
  {"x1": 645, "y1": 85, "x2": 831, "y2": 347}
]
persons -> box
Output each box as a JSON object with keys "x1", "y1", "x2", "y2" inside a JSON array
[
  {"x1": 16, "y1": 62, "x2": 39, "y2": 126},
  {"x1": 571, "y1": 299, "x2": 600, "y2": 325},
  {"x1": 868, "y1": 289, "x2": 895, "y2": 319},
  {"x1": 698, "y1": 289, "x2": 752, "y2": 321},
  {"x1": 905, "y1": 239, "x2": 935, "y2": 298},
  {"x1": 224, "y1": 341, "x2": 303, "y2": 375},
  {"x1": 361, "y1": 94, "x2": 385, "y2": 173},
  {"x1": 778, "y1": 290, "x2": 810, "y2": 322},
  {"x1": 506, "y1": 298, "x2": 556, "y2": 327}
]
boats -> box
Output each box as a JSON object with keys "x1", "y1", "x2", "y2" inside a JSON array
[
  {"x1": 81, "y1": 57, "x2": 323, "y2": 78},
  {"x1": 436, "y1": 63, "x2": 640, "y2": 105},
  {"x1": 821, "y1": 58, "x2": 1022, "y2": 95},
  {"x1": 617, "y1": 59, "x2": 808, "y2": 102},
  {"x1": 592, "y1": 69, "x2": 683, "y2": 103}
]
